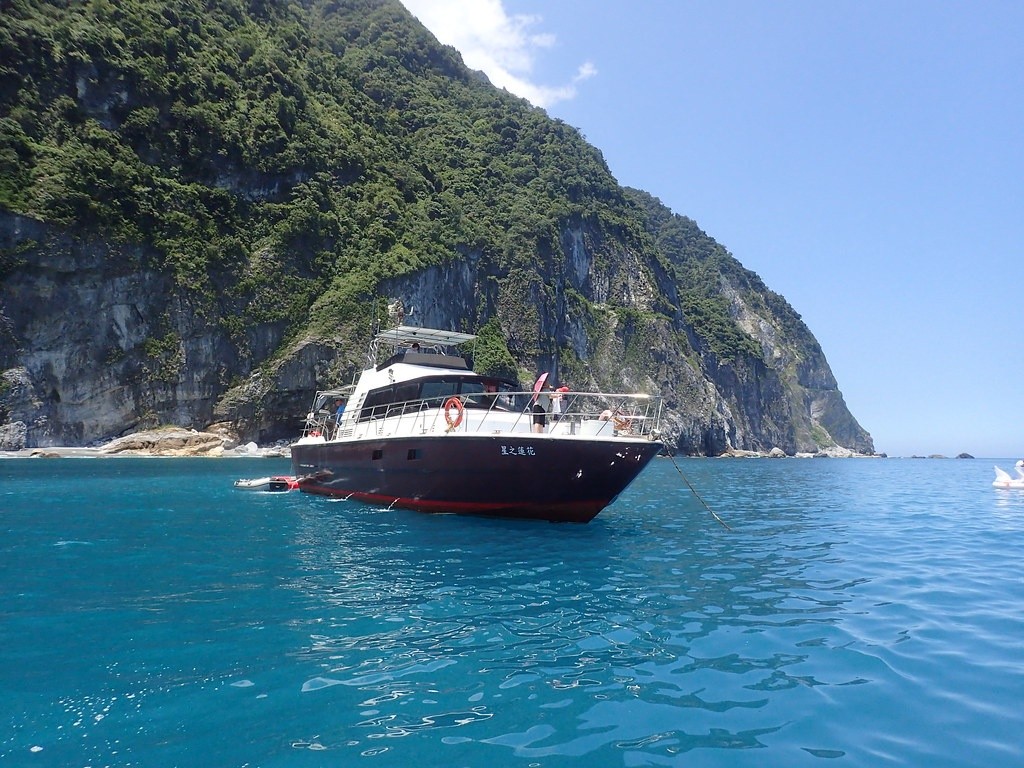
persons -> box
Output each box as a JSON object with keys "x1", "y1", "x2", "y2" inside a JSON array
[
  {"x1": 533, "y1": 381, "x2": 569, "y2": 433},
  {"x1": 412, "y1": 343, "x2": 419, "y2": 353},
  {"x1": 335, "y1": 398, "x2": 346, "y2": 427}
]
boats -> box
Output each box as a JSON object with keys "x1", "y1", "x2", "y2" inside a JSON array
[
  {"x1": 289, "y1": 299, "x2": 663, "y2": 524},
  {"x1": 233, "y1": 473, "x2": 300, "y2": 492}
]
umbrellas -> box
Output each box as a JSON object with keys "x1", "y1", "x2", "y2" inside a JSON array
[{"x1": 533, "y1": 373, "x2": 549, "y2": 402}]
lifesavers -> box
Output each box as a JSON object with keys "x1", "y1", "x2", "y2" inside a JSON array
[
  {"x1": 444, "y1": 397, "x2": 463, "y2": 428},
  {"x1": 600, "y1": 410, "x2": 613, "y2": 421}
]
